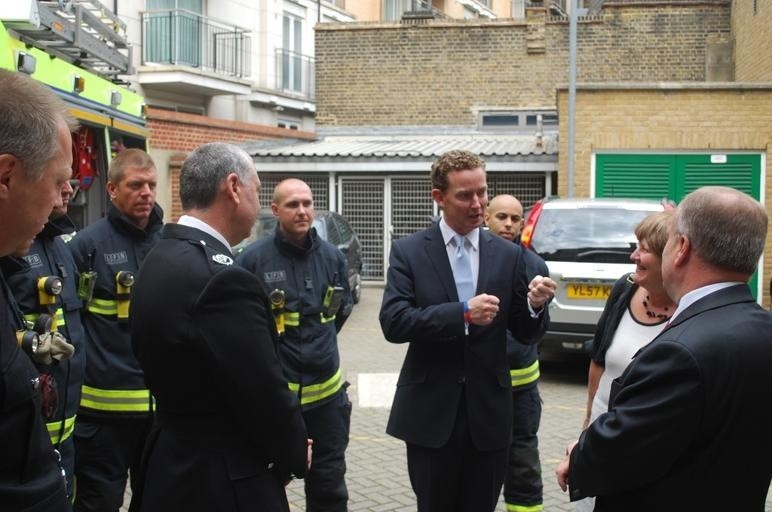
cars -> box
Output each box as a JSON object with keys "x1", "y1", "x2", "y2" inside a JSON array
[
  {"x1": 231, "y1": 208, "x2": 364, "y2": 302},
  {"x1": 521, "y1": 196, "x2": 675, "y2": 362}
]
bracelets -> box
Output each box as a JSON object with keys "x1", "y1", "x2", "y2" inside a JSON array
[{"x1": 463, "y1": 301, "x2": 471, "y2": 323}]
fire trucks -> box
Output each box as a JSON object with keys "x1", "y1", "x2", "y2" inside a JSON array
[{"x1": 0, "y1": 0, "x2": 151, "y2": 227}]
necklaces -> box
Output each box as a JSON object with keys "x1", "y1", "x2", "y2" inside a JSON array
[{"x1": 643, "y1": 295, "x2": 670, "y2": 319}]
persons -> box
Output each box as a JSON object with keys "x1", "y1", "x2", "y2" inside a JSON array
[
  {"x1": 377, "y1": 150, "x2": 557, "y2": 512},
  {"x1": 580, "y1": 211, "x2": 678, "y2": 430},
  {"x1": 233, "y1": 177, "x2": 355, "y2": 511},
  {"x1": 483, "y1": 194, "x2": 545, "y2": 512},
  {"x1": 128, "y1": 140, "x2": 315, "y2": 511},
  {"x1": 556, "y1": 184, "x2": 772, "y2": 512}
]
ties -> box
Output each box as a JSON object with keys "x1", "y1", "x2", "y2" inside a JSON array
[{"x1": 451, "y1": 234, "x2": 475, "y2": 302}]
